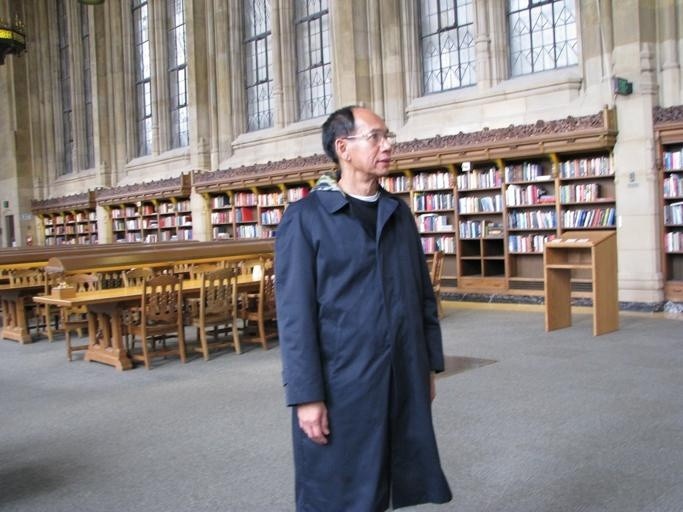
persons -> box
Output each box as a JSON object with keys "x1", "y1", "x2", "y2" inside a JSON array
[{"x1": 272, "y1": 105, "x2": 455, "y2": 512}]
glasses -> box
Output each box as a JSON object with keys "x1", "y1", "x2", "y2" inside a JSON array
[{"x1": 341, "y1": 132, "x2": 397, "y2": 146}]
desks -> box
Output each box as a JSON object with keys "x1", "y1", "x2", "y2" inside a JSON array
[
  {"x1": 0, "y1": 239, "x2": 276, "y2": 370},
  {"x1": 543, "y1": 230, "x2": 618, "y2": 337}
]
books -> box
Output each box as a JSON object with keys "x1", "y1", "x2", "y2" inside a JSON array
[
  {"x1": 377, "y1": 172, "x2": 455, "y2": 254},
  {"x1": 456, "y1": 167, "x2": 504, "y2": 239},
  {"x1": 557, "y1": 157, "x2": 616, "y2": 229},
  {"x1": 663, "y1": 152, "x2": 683, "y2": 253},
  {"x1": 504, "y1": 162, "x2": 558, "y2": 255},
  {"x1": 42, "y1": 187, "x2": 311, "y2": 247}
]
